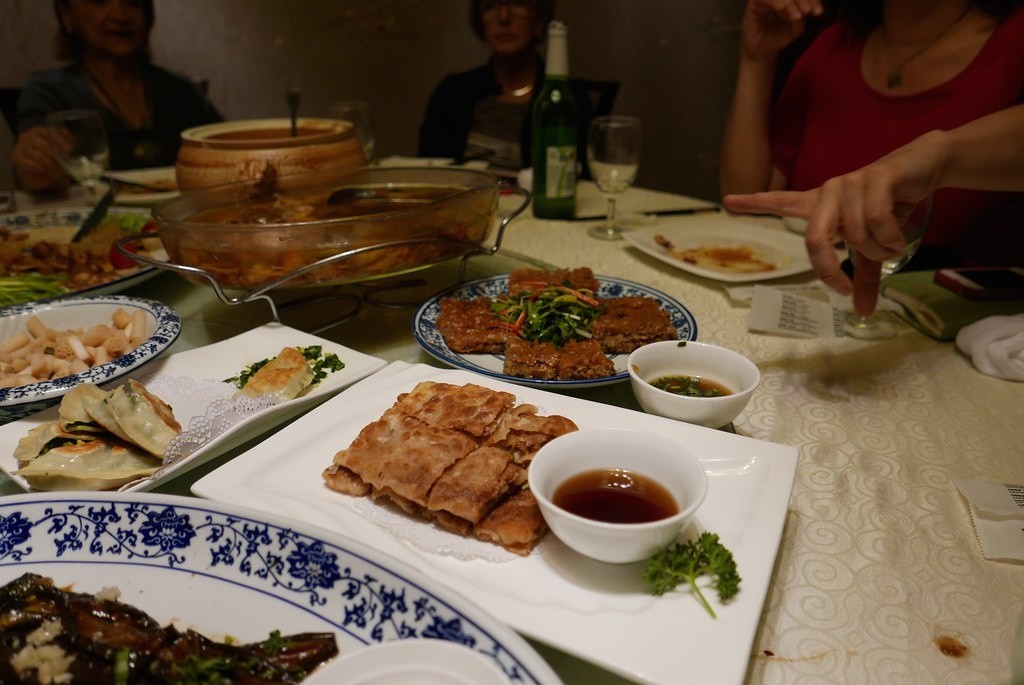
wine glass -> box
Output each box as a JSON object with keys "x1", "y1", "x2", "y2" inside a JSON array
[
  {"x1": 587, "y1": 116, "x2": 639, "y2": 240},
  {"x1": 46, "y1": 111, "x2": 109, "y2": 205},
  {"x1": 839, "y1": 192, "x2": 932, "y2": 341}
]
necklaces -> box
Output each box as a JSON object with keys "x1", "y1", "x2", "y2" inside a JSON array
[
  {"x1": 500, "y1": 79, "x2": 536, "y2": 98},
  {"x1": 86, "y1": 61, "x2": 158, "y2": 162},
  {"x1": 884, "y1": 4, "x2": 972, "y2": 87}
]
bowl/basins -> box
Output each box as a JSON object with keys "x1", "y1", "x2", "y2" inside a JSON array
[
  {"x1": 528, "y1": 428, "x2": 708, "y2": 564},
  {"x1": 175, "y1": 118, "x2": 368, "y2": 212},
  {"x1": 784, "y1": 215, "x2": 808, "y2": 235},
  {"x1": 151, "y1": 167, "x2": 498, "y2": 290},
  {"x1": 627, "y1": 341, "x2": 760, "y2": 428}
]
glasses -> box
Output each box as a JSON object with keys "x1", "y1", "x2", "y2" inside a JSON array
[{"x1": 479, "y1": 1, "x2": 535, "y2": 23}]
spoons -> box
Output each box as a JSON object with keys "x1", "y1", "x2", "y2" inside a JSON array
[{"x1": 98, "y1": 177, "x2": 169, "y2": 192}]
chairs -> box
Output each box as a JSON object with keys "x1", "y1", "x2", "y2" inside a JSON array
[{"x1": 574, "y1": 77, "x2": 622, "y2": 179}]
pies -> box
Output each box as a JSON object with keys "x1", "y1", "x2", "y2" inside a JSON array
[
  {"x1": 322, "y1": 380, "x2": 578, "y2": 555},
  {"x1": 502, "y1": 341, "x2": 616, "y2": 381},
  {"x1": 438, "y1": 296, "x2": 508, "y2": 352},
  {"x1": 505, "y1": 266, "x2": 599, "y2": 300},
  {"x1": 593, "y1": 296, "x2": 679, "y2": 353}
]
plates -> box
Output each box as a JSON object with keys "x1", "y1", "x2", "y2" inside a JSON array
[
  {"x1": 0, "y1": 297, "x2": 182, "y2": 406},
  {"x1": 301, "y1": 637, "x2": 510, "y2": 685},
  {"x1": 412, "y1": 271, "x2": 698, "y2": 388},
  {"x1": 0, "y1": 492, "x2": 564, "y2": 685},
  {"x1": 0, "y1": 322, "x2": 388, "y2": 491},
  {"x1": 191, "y1": 360, "x2": 799, "y2": 685},
  {"x1": 101, "y1": 168, "x2": 180, "y2": 203},
  {"x1": 0, "y1": 208, "x2": 170, "y2": 317},
  {"x1": 619, "y1": 222, "x2": 814, "y2": 281},
  {"x1": 378, "y1": 156, "x2": 453, "y2": 166}
]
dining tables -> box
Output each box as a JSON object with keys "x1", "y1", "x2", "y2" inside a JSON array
[{"x1": 132, "y1": 176, "x2": 1024, "y2": 685}]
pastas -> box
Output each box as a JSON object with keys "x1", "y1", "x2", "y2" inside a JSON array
[{"x1": 0, "y1": 308, "x2": 147, "y2": 389}]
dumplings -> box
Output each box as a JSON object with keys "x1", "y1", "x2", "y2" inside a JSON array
[
  {"x1": 10, "y1": 378, "x2": 182, "y2": 493},
  {"x1": 242, "y1": 348, "x2": 315, "y2": 399}
]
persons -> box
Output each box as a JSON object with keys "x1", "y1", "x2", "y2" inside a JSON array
[
  {"x1": 719, "y1": 0, "x2": 1024, "y2": 318},
  {"x1": 418, "y1": 0, "x2": 607, "y2": 181},
  {"x1": 0, "y1": 0, "x2": 228, "y2": 196}
]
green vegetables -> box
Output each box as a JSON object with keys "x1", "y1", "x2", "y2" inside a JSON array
[
  {"x1": 0, "y1": 272, "x2": 70, "y2": 310},
  {"x1": 650, "y1": 377, "x2": 718, "y2": 397},
  {"x1": 117, "y1": 629, "x2": 306, "y2": 685},
  {"x1": 221, "y1": 346, "x2": 345, "y2": 385},
  {"x1": 491, "y1": 280, "x2": 608, "y2": 347},
  {"x1": 642, "y1": 531, "x2": 741, "y2": 618}
]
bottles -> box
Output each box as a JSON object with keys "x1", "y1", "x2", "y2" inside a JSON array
[{"x1": 531, "y1": 19, "x2": 578, "y2": 219}]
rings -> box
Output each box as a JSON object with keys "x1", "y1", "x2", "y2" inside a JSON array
[{"x1": 22, "y1": 146, "x2": 33, "y2": 160}]
